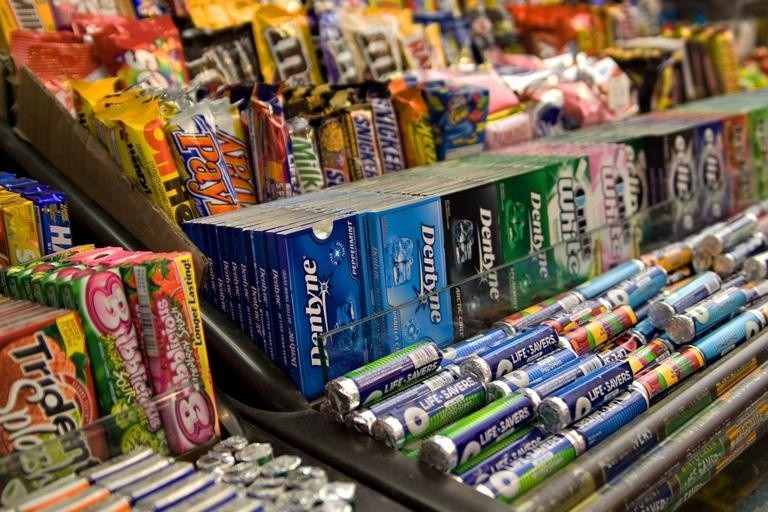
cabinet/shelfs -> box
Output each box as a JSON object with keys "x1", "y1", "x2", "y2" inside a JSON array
[{"x1": 1, "y1": 0, "x2": 768, "y2": 512}]
